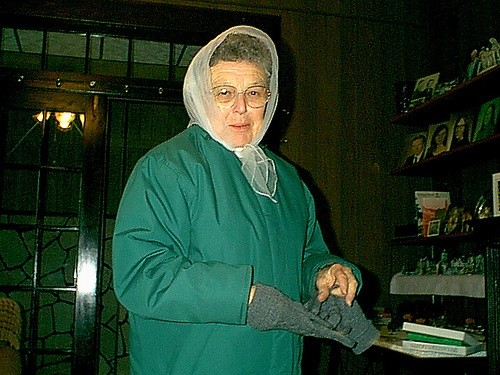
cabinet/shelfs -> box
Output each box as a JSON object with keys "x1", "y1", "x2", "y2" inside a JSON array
[{"x1": 371, "y1": 36, "x2": 500, "y2": 374}]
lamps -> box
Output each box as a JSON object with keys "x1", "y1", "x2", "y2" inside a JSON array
[{"x1": 33, "y1": 110, "x2": 85, "y2": 132}]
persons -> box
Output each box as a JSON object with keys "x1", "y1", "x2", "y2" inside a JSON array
[
  {"x1": 475, "y1": 104, "x2": 497, "y2": 140},
  {"x1": 423, "y1": 123, "x2": 450, "y2": 159},
  {"x1": 404, "y1": 41, "x2": 500, "y2": 105},
  {"x1": 112, "y1": 25, "x2": 364, "y2": 374},
  {"x1": 403, "y1": 135, "x2": 426, "y2": 166},
  {"x1": 449, "y1": 113, "x2": 470, "y2": 150}
]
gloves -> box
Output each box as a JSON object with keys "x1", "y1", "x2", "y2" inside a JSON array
[
  {"x1": 246, "y1": 281, "x2": 358, "y2": 348},
  {"x1": 306, "y1": 286, "x2": 380, "y2": 354}
]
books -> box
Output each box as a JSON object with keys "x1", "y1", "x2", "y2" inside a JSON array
[
  {"x1": 401, "y1": 321, "x2": 476, "y2": 346},
  {"x1": 402, "y1": 339, "x2": 484, "y2": 355},
  {"x1": 408, "y1": 332, "x2": 469, "y2": 347}
]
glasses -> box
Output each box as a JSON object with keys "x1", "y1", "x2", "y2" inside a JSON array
[{"x1": 212, "y1": 84, "x2": 272, "y2": 109}]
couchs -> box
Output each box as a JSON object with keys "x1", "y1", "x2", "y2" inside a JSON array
[{"x1": 0, "y1": 297, "x2": 22, "y2": 374}]
